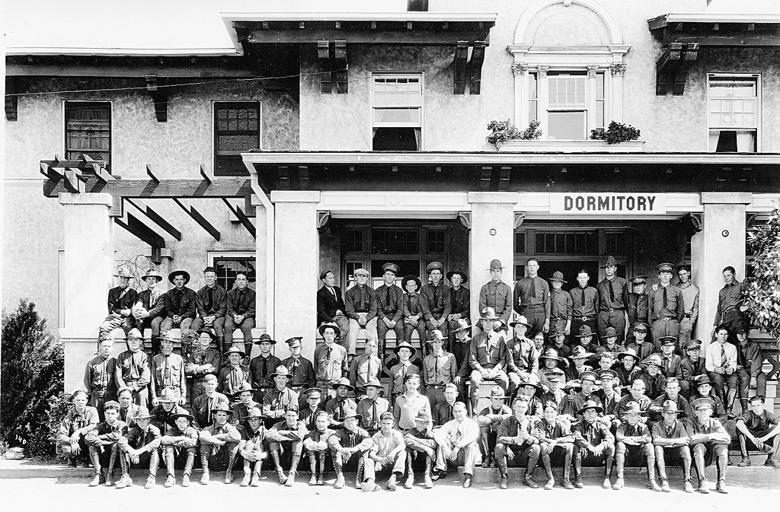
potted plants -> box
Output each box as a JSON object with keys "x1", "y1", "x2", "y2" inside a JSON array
[{"x1": 486, "y1": 117, "x2": 647, "y2": 152}]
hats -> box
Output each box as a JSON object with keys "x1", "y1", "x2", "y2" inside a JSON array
[
  {"x1": 321, "y1": 258, "x2": 468, "y2": 286},
  {"x1": 581, "y1": 287, "x2": 585, "y2": 309},
  {"x1": 392, "y1": 318, "x2": 473, "y2": 353},
  {"x1": 489, "y1": 259, "x2": 505, "y2": 269},
  {"x1": 546, "y1": 271, "x2": 567, "y2": 284},
  {"x1": 416, "y1": 408, "x2": 434, "y2": 423},
  {"x1": 600, "y1": 256, "x2": 621, "y2": 267},
  {"x1": 120, "y1": 325, "x2": 384, "y2": 421},
  {"x1": 676, "y1": 264, "x2": 688, "y2": 272},
  {"x1": 115, "y1": 267, "x2": 192, "y2": 284},
  {"x1": 479, "y1": 305, "x2": 714, "y2": 412},
  {"x1": 629, "y1": 275, "x2": 647, "y2": 282}
]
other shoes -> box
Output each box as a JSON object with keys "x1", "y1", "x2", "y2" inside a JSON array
[
  {"x1": 463, "y1": 476, "x2": 470, "y2": 489},
  {"x1": 360, "y1": 481, "x2": 382, "y2": 492},
  {"x1": 545, "y1": 478, "x2": 555, "y2": 489},
  {"x1": 500, "y1": 477, "x2": 508, "y2": 488},
  {"x1": 405, "y1": 476, "x2": 414, "y2": 488},
  {"x1": 698, "y1": 480, "x2": 709, "y2": 492},
  {"x1": 385, "y1": 479, "x2": 396, "y2": 490},
  {"x1": 89, "y1": 471, "x2": 362, "y2": 489},
  {"x1": 561, "y1": 477, "x2": 574, "y2": 488},
  {"x1": 431, "y1": 470, "x2": 444, "y2": 480},
  {"x1": 575, "y1": 475, "x2": 583, "y2": 488},
  {"x1": 602, "y1": 478, "x2": 611, "y2": 488},
  {"x1": 684, "y1": 481, "x2": 694, "y2": 491},
  {"x1": 423, "y1": 476, "x2": 432, "y2": 487},
  {"x1": 524, "y1": 477, "x2": 538, "y2": 488},
  {"x1": 648, "y1": 479, "x2": 659, "y2": 490},
  {"x1": 613, "y1": 477, "x2": 624, "y2": 489},
  {"x1": 716, "y1": 479, "x2": 727, "y2": 492},
  {"x1": 660, "y1": 478, "x2": 670, "y2": 491}
]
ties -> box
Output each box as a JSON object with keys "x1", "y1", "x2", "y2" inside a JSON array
[
  {"x1": 607, "y1": 279, "x2": 615, "y2": 301},
  {"x1": 720, "y1": 345, "x2": 728, "y2": 367},
  {"x1": 530, "y1": 277, "x2": 535, "y2": 298},
  {"x1": 663, "y1": 287, "x2": 668, "y2": 307}
]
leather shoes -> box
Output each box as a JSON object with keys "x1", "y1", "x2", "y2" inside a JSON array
[
  {"x1": 765, "y1": 453, "x2": 780, "y2": 468},
  {"x1": 738, "y1": 455, "x2": 752, "y2": 466}
]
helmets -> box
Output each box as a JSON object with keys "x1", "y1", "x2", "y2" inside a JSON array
[{"x1": 656, "y1": 261, "x2": 675, "y2": 274}]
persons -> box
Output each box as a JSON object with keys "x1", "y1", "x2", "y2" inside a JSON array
[
  {"x1": 295, "y1": 260, "x2": 512, "y2": 492},
  {"x1": 58, "y1": 255, "x2": 313, "y2": 488},
  {"x1": 494, "y1": 256, "x2": 780, "y2": 493}
]
